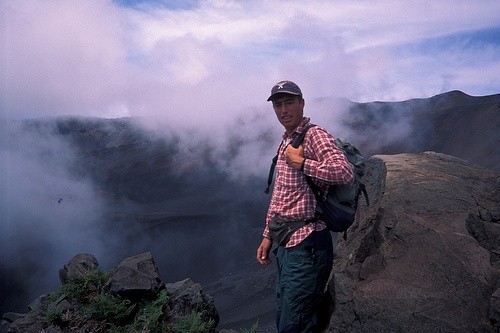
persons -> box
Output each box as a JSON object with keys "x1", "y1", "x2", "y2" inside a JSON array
[{"x1": 257, "y1": 80, "x2": 357, "y2": 333}]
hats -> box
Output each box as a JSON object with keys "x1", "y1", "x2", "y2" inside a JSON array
[{"x1": 267, "y1": 77, "x2": 303, "y2": 104}]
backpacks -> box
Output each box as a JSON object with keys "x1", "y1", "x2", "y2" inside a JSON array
[{"x1": 276, "y1": 122, "x2": 365, "y2": 232}]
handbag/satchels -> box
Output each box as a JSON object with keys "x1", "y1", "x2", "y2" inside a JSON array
[{"x1": 265, "y1": 215, "x2": 323, "y2": 249}]
